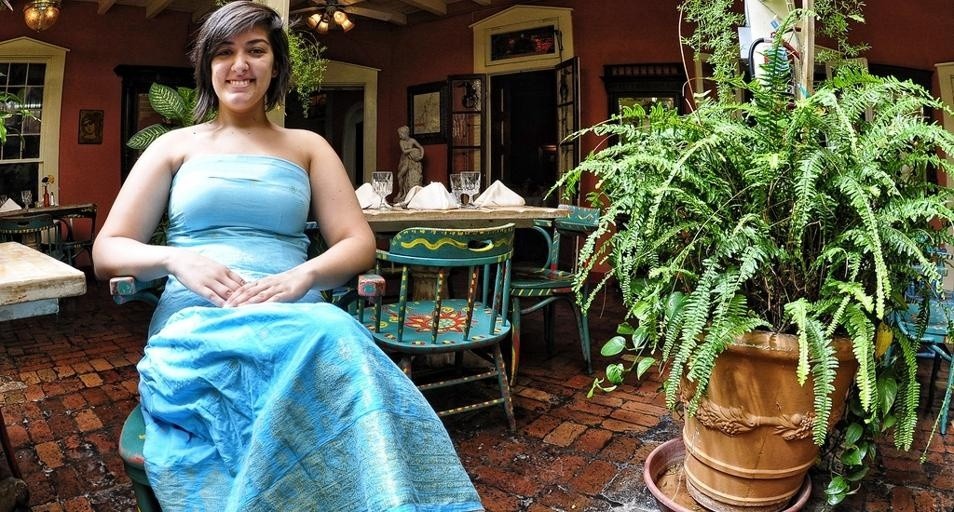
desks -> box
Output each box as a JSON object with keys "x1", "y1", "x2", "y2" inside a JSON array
[{"x1": 1, "y1": 241, "x2": 88, "y2": 479}]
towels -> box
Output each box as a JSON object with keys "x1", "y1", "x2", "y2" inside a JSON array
[
  {"x1": 401, "y1": 181, "x2": 458, "y2": 211},
  {"x1": 355, "y1": 182, "x2": 388, "y2": 211},
  {"x1": 472, "y1": 180, "x2": 526, "y2": 206},
  {"x1": 0, "y1": 197, "x2": 22, "y2": 212}
]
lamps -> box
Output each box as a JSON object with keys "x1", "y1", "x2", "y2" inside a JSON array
[
  {"x1": 22, "y1": 0, "x2": 62, "y2": 33},
  {"x1": 304, "y1": 0, "x2": 362, "y2": 34}
]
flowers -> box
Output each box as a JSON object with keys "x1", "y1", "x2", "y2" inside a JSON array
[{"x1": 41, "y1": 175, "x2": 55, "y2": 186}]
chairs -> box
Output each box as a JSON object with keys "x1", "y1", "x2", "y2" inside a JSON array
[
  {"x1": 5, "y1": 213, "x2": 74, "y2": 265},
  {"x1": 109, "y1": 221, "x2": 385, "y2": 511},
  {"x1": 341, "y1": 220, "x2": 518, "y2": 437},
  {"x1": 511, "y1": 204, "x2": 601, "y2": 388},
  {"x1": 64, "y1": 240, "x2": 101, "y2": 288},
  {"x1": 884, "y1": 246, "x2": 953, "y2": 436}
]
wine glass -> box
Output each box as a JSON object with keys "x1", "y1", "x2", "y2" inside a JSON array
[
  {"x1": 21, "y1": 190, "x2": 32, "y2": 211},
  {"x1": 461, "y1": 170, "x2": 481, "y2": 210},
  {"x1": 371, "y1": 171, "x2": 395, "y2": 211},
  {"x1": 450, "y1": 173, "x2": 461, "y2": 208}
]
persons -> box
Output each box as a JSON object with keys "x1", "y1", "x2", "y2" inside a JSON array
[
  {"x1": 91, "y1": 0, "x2": 488, "y2": 511},
  {"x1": 392, "y1": 126, "x2": 424, "y2": 202}
]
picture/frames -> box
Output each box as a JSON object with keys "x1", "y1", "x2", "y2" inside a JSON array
[
  {"x1": 407, "y1": 79, "x2": 449, "y2": 144},
  {"x1": 77, "y1": 108, "x2": 105, "y2": 145}
]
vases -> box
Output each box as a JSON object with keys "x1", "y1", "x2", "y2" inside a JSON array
[{"x1": 44, "y1": 186, "x2": 49, "y2": 207}]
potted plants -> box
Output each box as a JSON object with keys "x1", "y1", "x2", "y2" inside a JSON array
[{"x1": 542, "y1": 0, "x2": 954, "y2": 512}]
